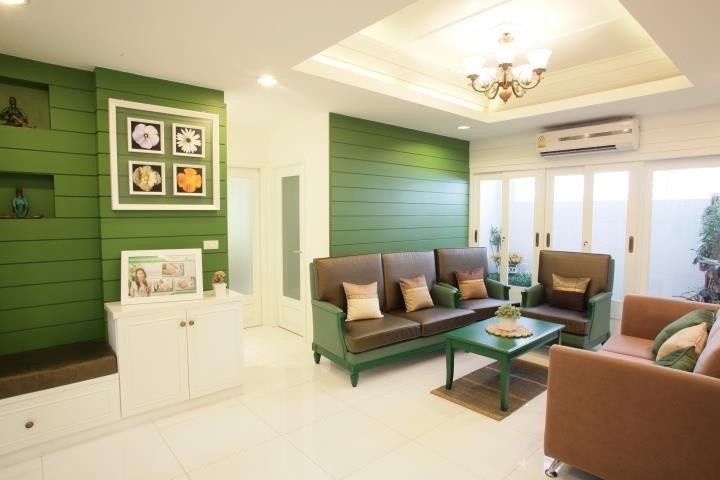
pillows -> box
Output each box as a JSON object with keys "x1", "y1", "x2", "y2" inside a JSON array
[
  {"x1": 551, "y1": 273, "x2": 591, "y2": 312},
  {"x1": 455, "y1": 267, "x2": 488, "y2": 300},
  {"x1": 342, "y1": 281, "x2": 384, "y2": 322},
  {"x1": 652, "y1": 309, "x2": 717, "y2": 372},
  {"x1": 399, "y1": 275, "x2": 434, "y2": 312}
]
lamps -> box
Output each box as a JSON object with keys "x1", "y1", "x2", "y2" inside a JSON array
[{"x1": 466, "y1": 33, "x2": 554, "y2": 105}]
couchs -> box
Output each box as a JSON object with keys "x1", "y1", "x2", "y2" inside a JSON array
[
  {"x1": 309, "y1": 247, "x2": 520, "y2": 386},
  {"x1": 544, "y1": 294, "x2": 720, "y2": 480}
]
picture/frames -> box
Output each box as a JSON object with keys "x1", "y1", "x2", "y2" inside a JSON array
[
  {"x1": 108, "y1": 98, "x2": 221, "y2": 210},
  {"x1": 121, "y1": 248, "x2": 203, "y2": 305}
]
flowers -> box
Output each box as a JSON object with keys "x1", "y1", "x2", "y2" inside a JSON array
[{"x1": 212, "y1": 271, "x2": 226, "y2": 283}]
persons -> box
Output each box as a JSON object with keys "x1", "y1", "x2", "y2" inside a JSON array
[
  {"x1": 128, "y1": 264, "x2": 192, "y2": 296},
  {"x1": 1, "y1": 187, "x2": 43, "y2": 218},
  {"x1": 0, "y1": 97, "x2": 35, "y2": 127}
]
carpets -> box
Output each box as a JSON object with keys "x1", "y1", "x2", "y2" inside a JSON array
[{"x1": 430, "y1": 358, "x2": 548, "y2": 422}]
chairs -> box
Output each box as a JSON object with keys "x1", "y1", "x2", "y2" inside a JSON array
[{"x1": 517, "y1": 250, "x2": 615, "y2": 350}]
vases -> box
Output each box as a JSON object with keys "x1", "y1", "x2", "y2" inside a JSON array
[{"x1": 212, "y1": 283, "x2": 227, "y2": 296}]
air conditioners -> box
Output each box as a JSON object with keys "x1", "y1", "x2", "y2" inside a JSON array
[{"x1": 535, "y1": 118, "x2": 640, "y2": 161}]
